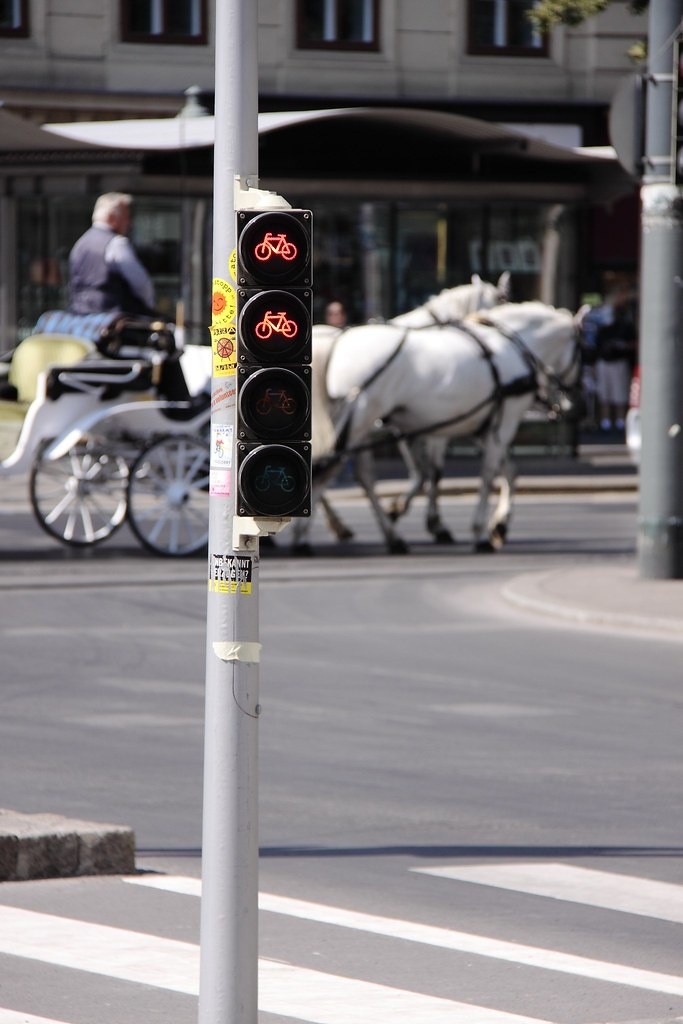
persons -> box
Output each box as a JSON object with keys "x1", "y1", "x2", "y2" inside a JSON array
[
  {"x1": 576, "y1": 282, "x2": 633, "y2": 432},
  {"x1": 68, "y1": 192, "x2": 211, "y2": 420},
  {"x1": 327, "y1": 303, "x2": 357, "y2": 488}
]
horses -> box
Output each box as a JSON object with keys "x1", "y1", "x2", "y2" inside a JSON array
[{"x1": 289, "y1": 270, "x2": 593, "y2": 554}]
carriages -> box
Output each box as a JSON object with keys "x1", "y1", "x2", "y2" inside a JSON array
[{"x1": 0, "y1": 271, "x2": 593, "y2": 558}]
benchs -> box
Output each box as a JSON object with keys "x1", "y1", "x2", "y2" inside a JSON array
[{"x1": 0, "y1": 332, "x2": 98, "y2": 422}]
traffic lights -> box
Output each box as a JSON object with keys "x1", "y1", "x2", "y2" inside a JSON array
[{"x1": 232, "y1": 207, "x2": 317, "y2": 521}]
